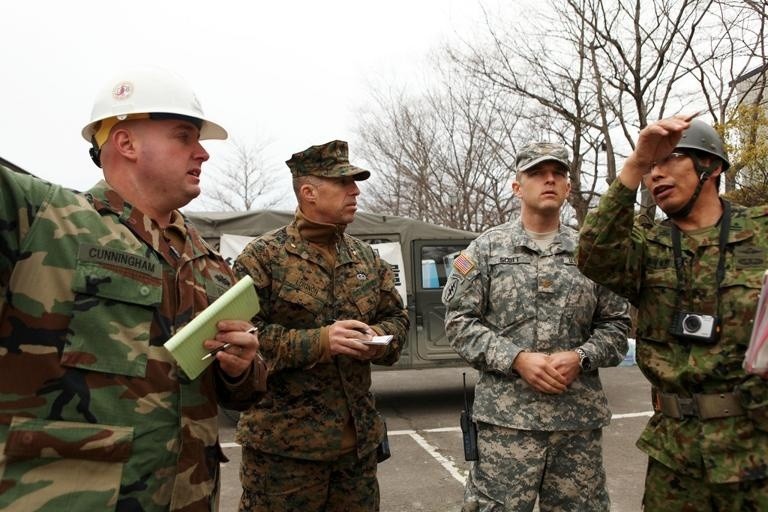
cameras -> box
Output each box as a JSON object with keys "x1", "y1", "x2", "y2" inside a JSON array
[{"x1": 670, "y1": 310, "x2": 715, "y2": 340}]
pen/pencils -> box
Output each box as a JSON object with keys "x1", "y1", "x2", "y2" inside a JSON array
[
  {"x1": 200, "y1": 327, "x2": 259, "y2": 360},
  {"x1": 328, "y1": 318, "x2": 372, "y2": 335}
]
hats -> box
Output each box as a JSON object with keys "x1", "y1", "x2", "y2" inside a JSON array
[
  {"x1": 285, "y1": 140, "x2": 370, "y2": 181},
  {"x1": 515, "y1": 142, "x2": 571, "y2": 173}
]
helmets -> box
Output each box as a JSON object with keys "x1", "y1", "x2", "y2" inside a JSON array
[
  {"x1": 79, "y1": 68, "x2": 229, "y2": 143},
  {"x1": 673, "y1": 116, "x2": 731, "y2": 172}
]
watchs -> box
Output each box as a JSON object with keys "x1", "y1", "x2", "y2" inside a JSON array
[{"x1": 573, "y1": 348, "x2": 591, "y2": 374}]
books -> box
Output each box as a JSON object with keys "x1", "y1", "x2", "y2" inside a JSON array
[{"x1": 163, "y1": 274, "x2": 260, "y2": 380}]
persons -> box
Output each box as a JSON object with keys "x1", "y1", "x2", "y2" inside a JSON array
[
  {"x1": 441, "y1": 141, "x2": 633, "y2": 512},
  {"x1": 232, "y1": 141, "x2": 410, "y2": 512},
  {"x1": 0, "y1": 66, "x2": 269, "y2": 512},
  {"x1": 577, "y1": 115, "x2": 768, "y2": 512}
]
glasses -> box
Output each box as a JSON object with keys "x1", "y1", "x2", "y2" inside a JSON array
[{"x1": 640, "y1": 150, "x2": 689, "y2": 175}]
